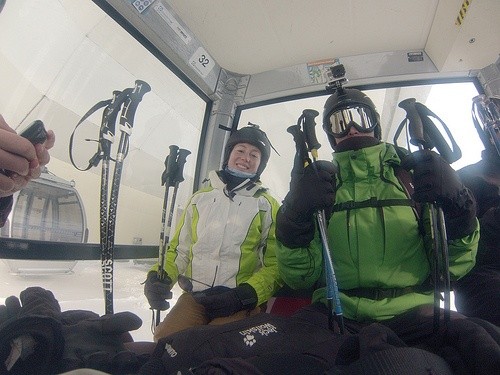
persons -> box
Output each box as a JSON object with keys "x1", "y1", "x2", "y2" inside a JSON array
[
  {"x1": 140, "y1": 127, "x2": 282, "y2": 344},
  {"x1": 146, "y1": 88, "x2": 500, "y2": 375},
  {"x1": 453, "y1": 94, "x2": 500, "y2": 325},
  {"x1": 0, "y1": 113, "x2": 55, "y2": 227}
]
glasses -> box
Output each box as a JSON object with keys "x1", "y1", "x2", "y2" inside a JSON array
[{"x1": 322, "y1": 104, "x2": 380, "y2": 137}]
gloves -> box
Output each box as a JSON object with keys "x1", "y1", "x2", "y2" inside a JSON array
[
  {"x1": 401, "y1": 150, "x2": 478, "y2": 241},
  {"x1": 143, "y1": 270, "x2": 173, "y2": 310},
  {"x1": 282, "y1": 160, "x2": 338, "y2": 219},
  {"x1": 60, "y1": 310, "x2": 142, "y2": 372},
  {"x1": 195, "y1": 282, "x2": 259, "y2": 320},
  {"x1": 0, "y1": 285, "x2": 64, "y2": 375}
]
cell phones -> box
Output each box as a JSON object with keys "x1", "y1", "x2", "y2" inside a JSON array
[{"x1": 0, "y1": 120, "x2": 48, "y2": 176}]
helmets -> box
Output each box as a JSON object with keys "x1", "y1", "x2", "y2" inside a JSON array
[
  {"x1": 219, "y1": 126, "x2": 271, "y2": 179},
  {"x1": 321, "y1": 88, "x2": 382, "y2": 150}
]
